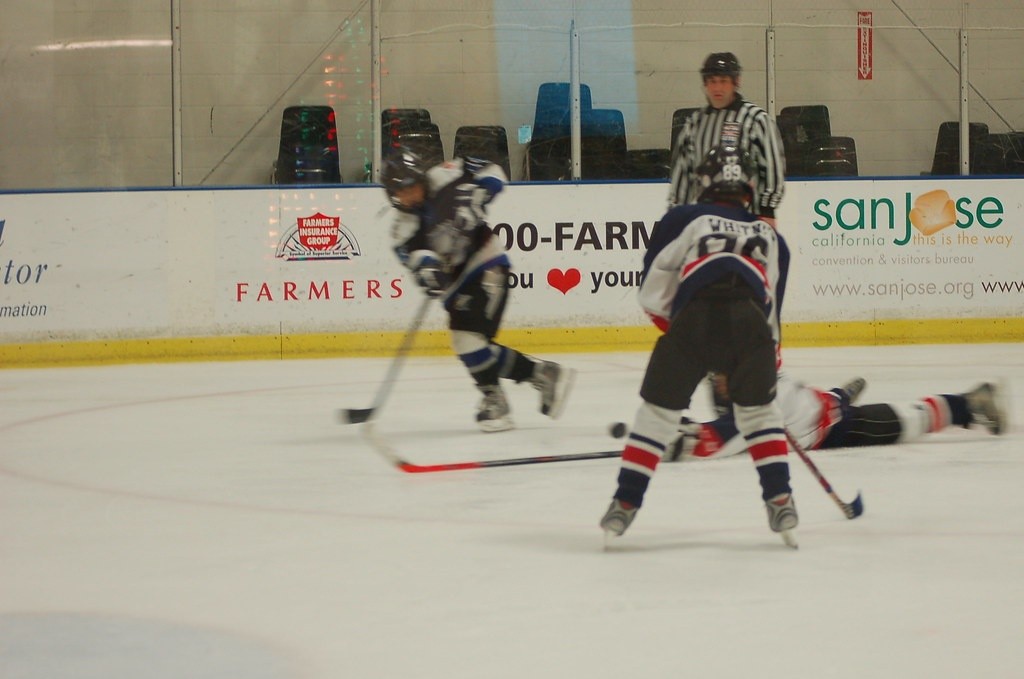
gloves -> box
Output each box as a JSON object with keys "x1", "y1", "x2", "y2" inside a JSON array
[
  {"x1": 455, "y1": 182, "x2": 490, "y2": 238},
  {"x1": 411, "y1": 249, "x2": 448, "y2": 298}
]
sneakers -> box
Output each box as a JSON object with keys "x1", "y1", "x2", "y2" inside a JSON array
[
  {"x1": 962, "y1": 384, "x2": 1007, "y2": 436},
  {"x1": 601, "y1": 499, "x2": 639, "y2": 551},
  {"x1": 475, "y1": 382, "x2": 517, "y2": 432},
  {"x1": 844, "y1": 377, "x2": 867, "y2": 405},
  {"x1": 516, "y1": 360, "x2": 576, "y2": 428},
  {"x1": 765, "y1": 493, "x2": 799, "y2": 549}
]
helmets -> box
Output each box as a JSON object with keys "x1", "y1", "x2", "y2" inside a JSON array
[
  {"x1": 700, "y1": 51, "x2": 742, "y2": 76},
  {"x1": 384, "y1": 152, "x2": 429, "y2": 215},
  {"x1": 696, "y1": 179, "x2": 754, "y2": 215}
]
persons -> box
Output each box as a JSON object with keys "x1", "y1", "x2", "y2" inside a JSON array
[
  {"x1": 598, "y1": 186, "x2": 800, "y2": 551},
  {"x1": 657, "y1": 50, "x2": 1006, "y2": 465},
  {"x1": 380, "y1": 153, "x2": 575, "y2": 435}
]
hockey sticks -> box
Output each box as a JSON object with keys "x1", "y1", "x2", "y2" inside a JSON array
[
  {"x1": 784, "y1": 419, "x2": 863, "y2": 519},
  {"x1": 368, "y1": 426, "x2": 625, "y2": 474},
  {"x1": 335, "y1": 222, "x2": 465, "y2": 423}
]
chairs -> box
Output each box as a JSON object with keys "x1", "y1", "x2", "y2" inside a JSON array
[
  {"x1": 271, "y1": 105, "x2": 344, "y2": 184},
  {"x1": 524, "y1": 83, "x2": 703, "y2": 180},
  {"x1": 920, "y1": 121, "x2": 1024, "y2": 175},
  {"x1": 382, "y1": 110, "x2": 511, "y2": 181},
  {"x1": 776, "y1": 105, "x2": 858, "y2": 177}
]
